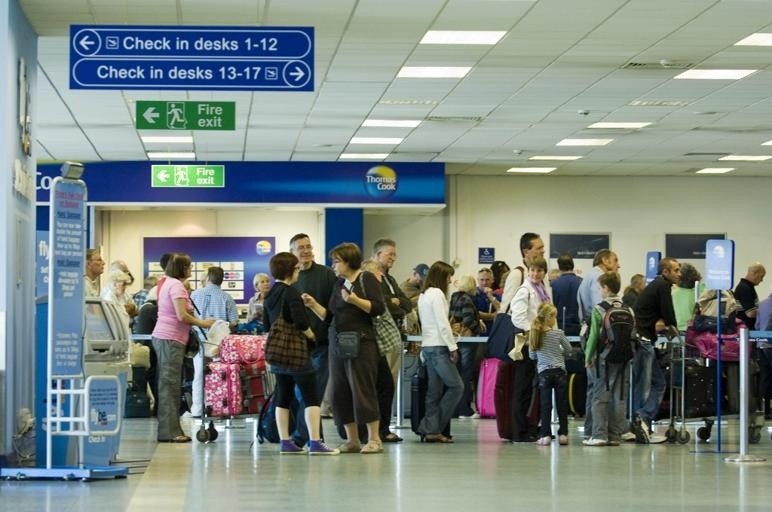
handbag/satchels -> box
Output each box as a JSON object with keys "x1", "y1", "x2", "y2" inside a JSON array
[
  {"x1": 131, "y1": 344, "x2": 151, "y2": 370},
  {"x1": 372, "y1": 310, "x2": 401, "y2": 357},
  {"x1": 449, "y1": 321, "x2": 472, "y2": 336},
  {"x1": 122, "y1": 380, "x2": 152, "y2": 418},
  {"x1": 265, "y1": 318, "x2": 309, "y2": 371},
  {"x1": 332, "y1": 331, "x2": 360, "y2": 361},
  {"x1": 484, "y1": 314, "x2": 525, "y2": 362},
  {"x1": 222, "y1": 333, "x2": 268, "y2": 366},
  {"x1": 185, "y1": 328, "x2": 199, "y2": 360}
]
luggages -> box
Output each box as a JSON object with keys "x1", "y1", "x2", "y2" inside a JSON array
[
  {"x1": 495, "y1": 362, "x2": 541, "y2": 439},
  {"x1": 395, "y1": 355, "x2": 451, "y2": 435},
  {"x1": 239, "y1": 360, "x2": 275, "y2": 414},
  {"x1": 476, "y1": 358, "x2": 502, "y2": 418},
  {"x1": 568, "y1": 369, "x2": 585, "y2": 419},
  {"x1": 204, "y1": 357, "x2": 243, "y2": 416}
]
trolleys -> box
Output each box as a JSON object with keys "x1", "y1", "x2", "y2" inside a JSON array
[
  {"x1": 663, "y1": 323, "x2": 769, "y2": 446},
  {"x1": 183, "y1": 300, "x2": 276, "y2": 443}
]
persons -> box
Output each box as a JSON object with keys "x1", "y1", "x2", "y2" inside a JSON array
[{"x1": 85, "y1": 233, "x2": 772, "y2": 455}]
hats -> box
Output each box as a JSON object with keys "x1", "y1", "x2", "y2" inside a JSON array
[
  {"x1": 107, "y1": 269, "x2": 131, "y2": 281},
  {"x1": 416, "y1": 264, "x2": 430, "y2": 276}
]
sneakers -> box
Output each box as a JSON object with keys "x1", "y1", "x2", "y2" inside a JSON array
[
  {"x1": 559, "y1": 434, "x2": 567, "y2": 444},
  {"x1": 582, "y1": 412, "x2": 669, "y2": 446},
  {"x1": 279, "y1": 441, "x2": 307, "y2": 454},
  {"x1": 158, "y1": 436, "x2": 190, "y2": 442},
  {"x1": 536, "y1": 435, "x2": 551, "y2": 445},
  {"x1": 381, "y1": 432, "x2": 398, "y2": 441},
  {"x1": 459, "y1": 413, "x2": 480, "y2": 420},
  {"x1": 308, "y1": 439, "x2": 340, "y2": 455}
]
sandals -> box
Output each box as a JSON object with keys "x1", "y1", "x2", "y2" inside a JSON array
[
  {"x1": 337, "y1": 440, "x2": 361, "y2": 452},
  {"x1": 359, "y1": 440, "x2": 384, "y2": 453},
  {"x1": 425, "y1": 434, "x2": 453, "y2": 443}
]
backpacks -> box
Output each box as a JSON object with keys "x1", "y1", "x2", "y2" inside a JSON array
[
  {"x1": 595, "y1": 301, "x2": 637, "y2": 364},
  {"x1": 685, "y1": 288, "x2": 752, "y2": 362},
  {"x1": 256, "y1": 389, "x2": 299, "y2": 444}
]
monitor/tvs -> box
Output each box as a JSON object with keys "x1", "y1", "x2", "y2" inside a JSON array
[{"x1": 84, "y1": 301, "x2": 116, "y2": 341}]
